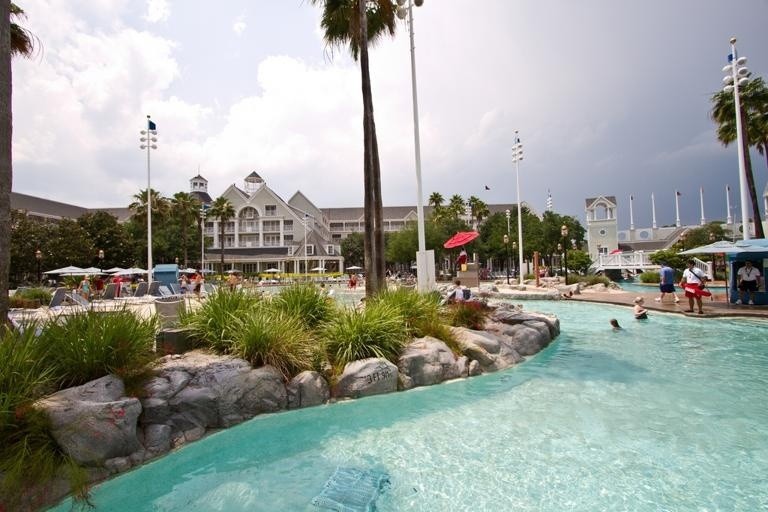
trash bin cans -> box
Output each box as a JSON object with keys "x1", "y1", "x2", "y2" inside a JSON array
[{"x1": 155, "y1": 295, "x2": 186, "y2": 327}]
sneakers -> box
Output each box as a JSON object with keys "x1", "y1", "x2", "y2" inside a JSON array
[
  {"x1": 748, "y1": 300, "x2": 753, "y2": 305},
  {"x1": 654, "y1": 298, "x2": 663, "y2": 303},
  {"x1": 735, "y1": 299, "x2": 742, "y2": 305},
  {"x1": 672, "y1": 297, "x2": 679, "y2": 303}
]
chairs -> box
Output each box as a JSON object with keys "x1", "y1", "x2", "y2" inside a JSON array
[{"x1": 21, "y1": 281, "x2": 217, "y2": 311}]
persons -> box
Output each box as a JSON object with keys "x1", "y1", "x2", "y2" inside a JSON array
[
  {"x1": 609, "y1": 319, "x2": 622, "y2": 330},
  {"x1": 633, "y1": 296, "x2": 648, "y2": 319},
  {"x1": 656, "y1": 260, "x2": 679, "y2": 302},
  {"x1": 679, "y1": 260, "x2": 712, "y2": 314},
  {"x1": 319, "y1": 268, "x2": 463, "y2": 311},
  {"x1": 192, "y1": 271, "x2": 201, "y2": 302},
  {"x1": 455, "y1": 248, "x2": 467, "y2": 270},
  {"x1": 78, "y1": 275, "x2": 93, "y2": 300},
  {"x1": 736, "y1": 260, "x2": 761, "y2": 305},
  {"x1": 230, "y1": 272, "x2": 237, "y2": 292}
]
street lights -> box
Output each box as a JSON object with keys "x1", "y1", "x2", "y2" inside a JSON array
[
  {"x1": 464, "y1": 200, "x2": 473, "y2": 226},
  {"x1": 174, "y1": 256, "x2": 180, "y2": 265},
  {"x1": 722, "y1": 38, "x2": 750, "y2": 241},
  {"x1": 34, "y1": 250, "x2": 43, "y2": 290},
  {"x1": 504, "y1": 130, "x2": 524, "y2": 290},
  {"x1": 557, "y1": 225, "x2": 578, "y2": 286},
  {"x1": 139, "y1": 115, "x2": 157, "y2": 294},
  {"x1": 199, "y1": 202, "x2": 207, "y2": 279},
  {"x1": 301, "y1": 213, "x2": 310, "y2": 283},
  {"x1": 546, "y1": 189, "x2": 553, "y2": 212},
  {"x1": 98, "y1": 249, "x2": 105, "y2": 272},
  {"x1": 396, "y1": 0, "x2": 427, "y2": 297}
]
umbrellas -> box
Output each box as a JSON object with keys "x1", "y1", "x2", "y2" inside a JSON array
[
  {"x1": 224, "y1": 269, "x2": 243, "y2": 274},
  {"x1": 263, "y1": 268, "x2": 283, "y2": 273},
  {"x1": 675, "y1": 237, "x2": 768, "y2": 304},
  {"x1": 41, "y1": 264, "x2": 216, "y2": 294},
  {"x1": 345, "y1": 265, "x2": 363, "y2": 270},
  {"x1": 311, "y1": 267, "x2": 328, "y2": 271},
  {"x1": 443, "y1": 231, "x2": 479, "y2": 249}
]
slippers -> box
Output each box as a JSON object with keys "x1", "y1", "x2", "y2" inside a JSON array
[
  {"x1": 684, "y1": 310, "x2": 693, "y2": 312},
  {"x1": 698, "y1": 311, "x2": 702, "y2": 314}
]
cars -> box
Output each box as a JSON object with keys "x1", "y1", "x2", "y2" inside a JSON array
[{"x1": 539, "y1": 266, "x2": 571, "y2": 278}]
